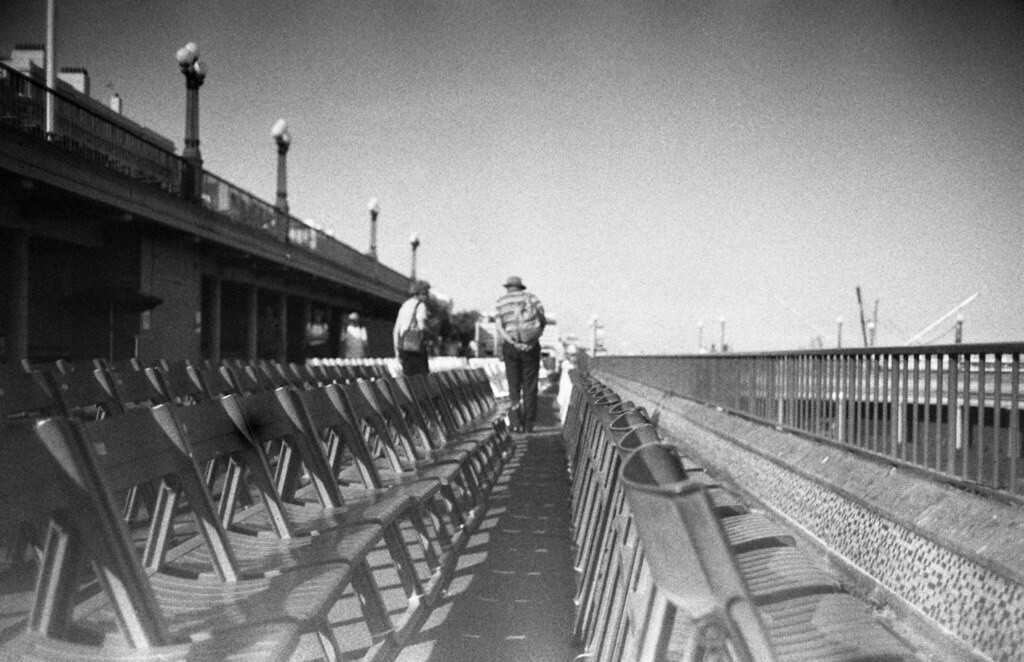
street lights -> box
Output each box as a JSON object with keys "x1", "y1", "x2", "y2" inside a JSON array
[
  {"x1": 367, "y1": 197, "x2": 380, "y2": 263},
  {"x1": 698, "y1": 320, "x2": 704, "y2": 354},
  {"x1": 955, "y1": 313, "x2": 963, "y2": 344},
  {"x1": 867, "y1": 319, "x2": 876, "y2": 346},
  {"x1": 588, "y1": 317, "x2": 605, "y2": 359},
  {"x1": 174, "y1": 39, "x2": 205, "y2": 166},
  {"x1": 836, "y1": 315, "x2": 843, "y2": 348},
  {"x1": 719, "y1": 314, "x2": 726, "y2": 354},
  {"x1": 409, "y1": 230, "x2": 419, "y2": 281},
  {"x1": 271, "y1": 116, "x2": 292, "y2": 217}
]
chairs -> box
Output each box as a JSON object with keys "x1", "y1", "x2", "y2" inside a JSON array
[{"x1": 0, "y1": 354, "x2": 923, "y2": 662}]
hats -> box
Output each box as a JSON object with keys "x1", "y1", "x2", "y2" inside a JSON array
[
  {"x1": 314, "y1": 308, "x2": 323, "y2": 316},
  {"x1": 347, "y1": 312, "x2": 360, "y2": 321},
  {"x1": 503, "y1": 276, "x2": 526, "y2": 290},
  {"x1": 408, "y1": 280, "x2": 430, "y2": 294}
]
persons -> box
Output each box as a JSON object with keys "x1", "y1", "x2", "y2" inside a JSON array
[
  {"x1": 303, "y1": 309, "x2": 330, "y2": 358},
  {"x1": 339, "y1": 312, "x2": 368, "y2": 358},
  {"x1": 494, "y1": 276, "x2": 546, "y2": 435},
  {"x1": 392, "y1": 281, "x2": 431, "y2": 375}
]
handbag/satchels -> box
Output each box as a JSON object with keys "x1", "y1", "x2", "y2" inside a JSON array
[
  {"x1": 518, "y1": 292, "x2": 541, "y2": 342},
  {"x1": 397, "y1": 330, "x2": 422, "y2": 352}
]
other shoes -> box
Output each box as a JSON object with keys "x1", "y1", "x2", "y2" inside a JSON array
[
  {"x1": 524, "y1": 426, "x2": 533, "y2": 432},
  {"x1": 512, "y1": 426, "x2": 524, "y2": 432}
]
